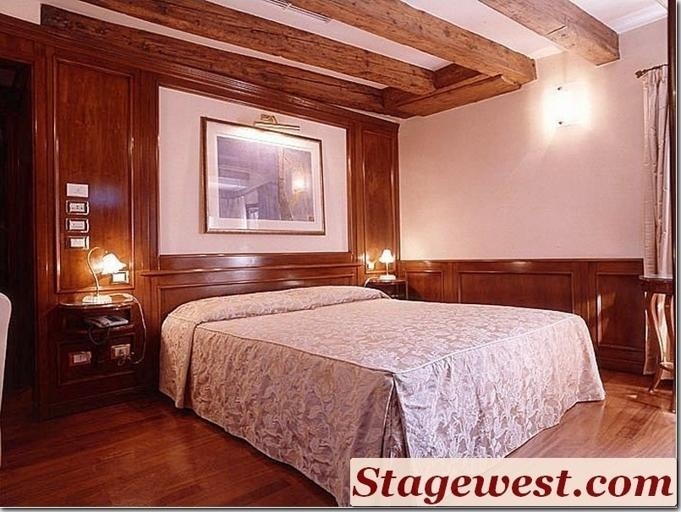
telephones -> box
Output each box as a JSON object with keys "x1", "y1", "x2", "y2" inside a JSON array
[{"x1": 84, "y1": 314, "x2": 130, "y2": 346}]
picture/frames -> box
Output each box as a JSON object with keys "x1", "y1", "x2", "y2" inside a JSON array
[{"x1": 200, "y1": 116, "x2": 326, "y2": 236}]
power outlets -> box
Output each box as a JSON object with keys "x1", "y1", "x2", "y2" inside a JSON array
[
  {"x1": 70, "y1": 221, "x2": 84, "y2": 230},
  {"x1": 69, "y1": 202, "x2": 87, "y2": 212}
]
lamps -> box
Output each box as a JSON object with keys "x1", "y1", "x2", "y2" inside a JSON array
[
  {"x1": 379, "y1": 248, "x2": 397, "y2": 280},
  {"x1": 83, "y1": 247, "x2": 126, "y2": 304},
  {"x1": 254, "y1": 114, "x2": 300, "y2": 131}
]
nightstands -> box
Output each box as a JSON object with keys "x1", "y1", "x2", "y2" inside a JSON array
[
  {"x1": 35, "y1": 301, "x2": 141, "y2": 422},
  {"x1": 366, "y1": 278, "x2": 407, "y2": 301}
]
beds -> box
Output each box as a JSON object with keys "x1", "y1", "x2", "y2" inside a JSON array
[
  {"x1": 148, "y1": 254, "x2": 583, "y2": 483},
  {"x1": 641, "y1": 275, "x2": 677, "y2": 413}
]
